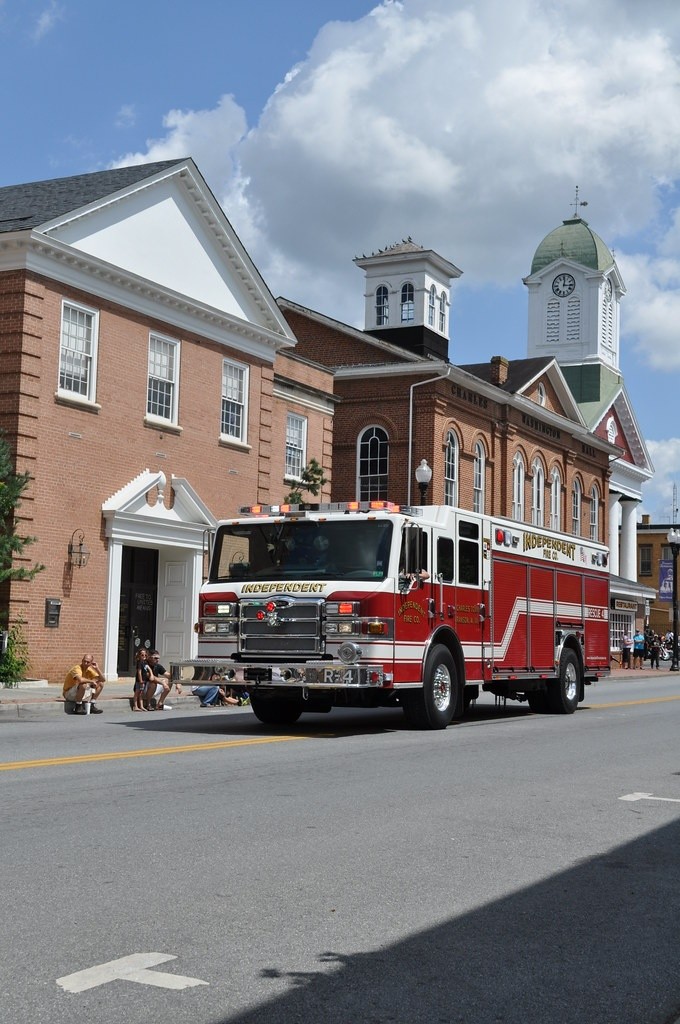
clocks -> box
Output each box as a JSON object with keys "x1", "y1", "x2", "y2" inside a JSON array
[
  {"x1": 605, "y1": 278, "x2": 612, "y2": 302},
  {"x1": 552, "y1": 273, "x2": 576, "y2": 298}
]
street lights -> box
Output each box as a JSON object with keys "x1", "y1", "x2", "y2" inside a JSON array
[
  {"x1": 666, "y1": 527, "x2": 680, "y2": 671},
  {"x1": 415, "y1": 457, "x2": 432, "y2": 505}
]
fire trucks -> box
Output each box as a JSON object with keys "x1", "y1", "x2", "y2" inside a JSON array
[{"x1": 166, "y1": 502, "x2": 621, "y2": 729}]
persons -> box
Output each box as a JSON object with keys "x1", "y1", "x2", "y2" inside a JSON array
[
  {"x1": 634, "y1": 630, "x2": 645, "y2": 670},
  {"x1": 63, "y1": 654, "x2": 106, "y2": 714},
  {"x1": 132, "y1": 648, "x2": 182, "y2": 712},
  {"x1": 645, "y1": 625, "x2": 674, "y2": 670},
  {"x1": 193, "y1": 675, "x2": 250, "y2": 708},
  {"x1": 622, "y1": 629, "x2": 633, "y2": 669},
  {"x1": 409, "y1": 568, "x2": 430, "y2": 581}
]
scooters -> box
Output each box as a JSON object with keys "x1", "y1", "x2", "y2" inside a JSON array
[{"x1": 646, "y1": 641, "x2": 671, "y2": 661}]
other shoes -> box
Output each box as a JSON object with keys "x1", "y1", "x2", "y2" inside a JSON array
[
  {"x1": 133, "y1": 707, "x2": 141, "y2": 711},
  {"x1": 140, "y1": 708, "x2": 147, "y2": 711},
  {"x1": 201, "y1": 703, "x2": 212, "y2": 707},
  {"x1": 146, "y1": 707, "x2": 154, "y2": 710},
  {"x1": 157, "y1": 706, "x2": 163, "y2": 710}
]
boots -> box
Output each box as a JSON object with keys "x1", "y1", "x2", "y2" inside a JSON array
[
  {"x1": 89, "y1": 702, "x2": 103, "y2": 714},
  {"x1": 75, "y1": 702, "x2": 87, "y2": 714}
]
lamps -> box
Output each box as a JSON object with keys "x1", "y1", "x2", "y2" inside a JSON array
[{"x1": 65, "y1": 529, "x2": 91, "y2": 572}]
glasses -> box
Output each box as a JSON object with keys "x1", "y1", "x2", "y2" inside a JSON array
[{"x1": 151, "y1": 656, "x2": 159, "y2": 660}]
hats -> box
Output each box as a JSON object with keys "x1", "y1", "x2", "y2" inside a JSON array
[{"x1": 635, "y1": 629, "x2": 639, "y2": 632}]
